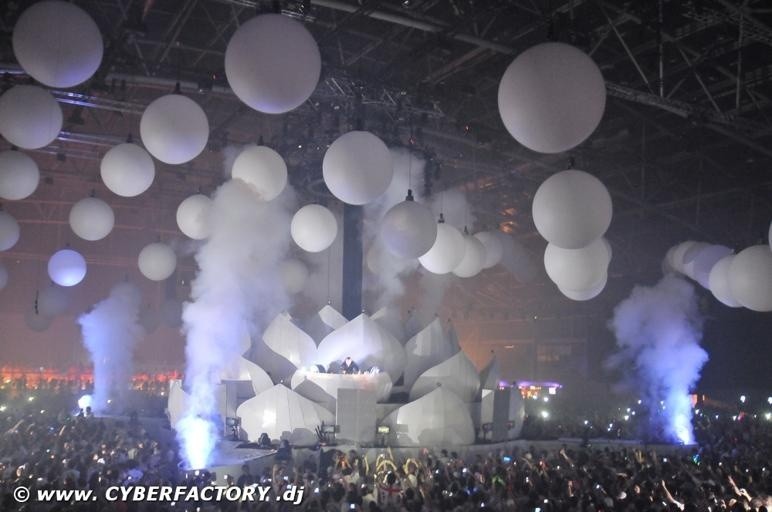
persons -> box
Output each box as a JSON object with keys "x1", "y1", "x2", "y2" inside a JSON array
[{"x1": 2, "y1": 355, "x2": 771, "y2": 511}]
